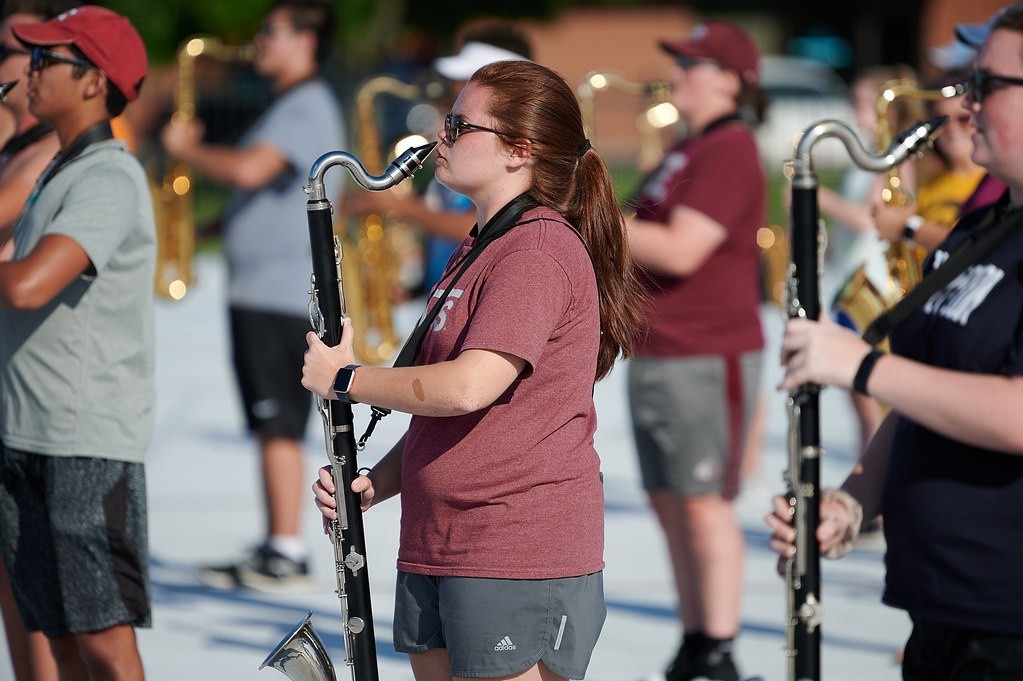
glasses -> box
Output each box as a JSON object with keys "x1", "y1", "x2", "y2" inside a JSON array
[
  {"x1": 0, "y1": 43, "x2": 29, "y2": 67},
  {"x1": 443, "y1": 113, "x2": 517, "y2": 148},
  {"x1": 676, "y1": 52, "x2": 726, "y2": 70},
  {"x1": 969, "y1": 68, "x2": 1023, "y2": 102},
  {"x1": 30, "y1": 48, "x2": 96, "y2": 72}
]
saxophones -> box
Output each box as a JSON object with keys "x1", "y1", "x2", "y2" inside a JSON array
[
  {"x1": 257, "y1": 139, "x2": 439, "y2": 681},
  {"x1": 141, "y1": 36, "x2": 262, "y2": 303},
  {"x1": 827, "y1": 75, "x2": 974, "y2": 423},
  {"x1": 741, "y1": 114, "x2": 953, "y2": 681},
  {"x1": 573, "y1": 69, "x2": 675, "y2": 154},
  {"x1": 329, "y1": 75, "x2": 449, "y2": 367}
]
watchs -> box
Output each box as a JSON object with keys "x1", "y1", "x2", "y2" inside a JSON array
[
  {"x1": 333, "y1": 364, "x2": 361, "y2": 404},
  {"x1": 899, "y1": 214, "x2": 925, "y2": 246}
]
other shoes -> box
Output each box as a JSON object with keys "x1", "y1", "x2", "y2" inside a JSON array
[
  {"x1": 195, "y1": 539, "x2": 309, "y2": 593},
  {"x1": 663, "y1": 629, "x2": 740, "y2": 681}
]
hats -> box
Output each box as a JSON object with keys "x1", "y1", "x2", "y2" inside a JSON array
[
  {"x1": 11, "y1": 7, "x2": 148, "y2": 102},
  {"x1": 656, "y1": 23, "x2": 760, "y2": 89},
  {"x1": 434, "y1": 42, "x2": 535, "y2": 81},
  {"x1": 929, "y1": 40, "x2": 980, "y2": 72},
  {"x1": 954, "y1": 10, "x2": 1008, "y2": 50}
]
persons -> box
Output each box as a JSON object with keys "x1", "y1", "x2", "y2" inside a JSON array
[
  {"x1": 0, "y1": 0, "x2": 158, "y2": 681},
  {"x1": 347, "y1": 16, "x2": 533, "y2": 298},
  {"x1": 300, "y1": 58, "x2": 646, "y2": 681},
  {"x1": 610, "y1": 23, "x2": 769, "y2": 681},
  {"x1": 764, "y1": 3, "x2": 1023, "y2": 681},
  {"x1": 160, "y1": 0, "x2": 349, "y2": 593}
]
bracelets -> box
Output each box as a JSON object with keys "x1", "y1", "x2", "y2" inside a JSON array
[
  {"x1": 854, "y1": 350, "x2": 887, "y2": 395},
  {"x1": 822, "y1": 489, "x2": 864, "y2": 558}
]
